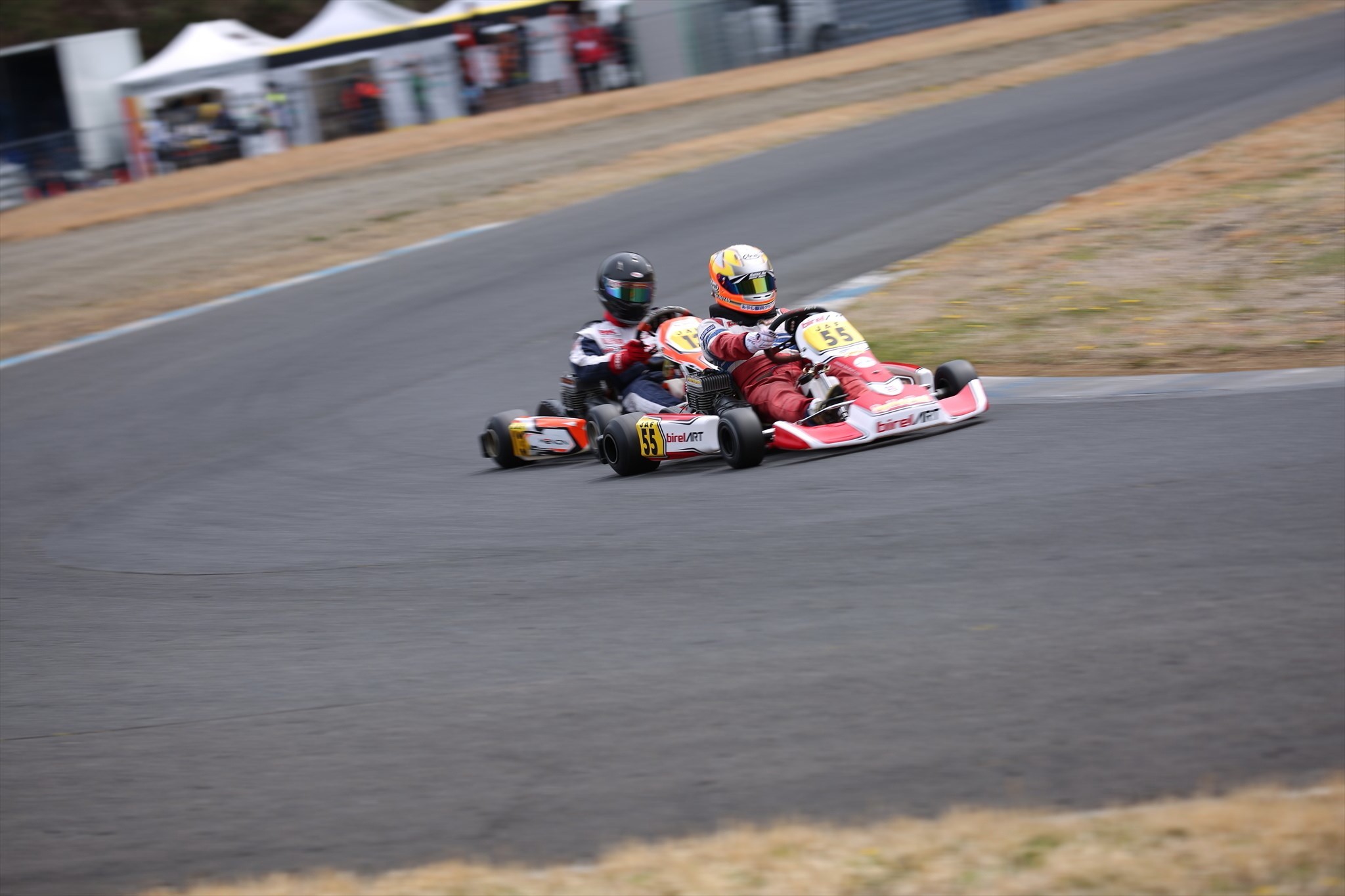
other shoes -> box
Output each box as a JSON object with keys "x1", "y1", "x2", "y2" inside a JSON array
[{"x1": 807, "y1": 384, "x2": 845, "y2": 425}]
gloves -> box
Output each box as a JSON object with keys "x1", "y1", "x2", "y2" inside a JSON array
[
  {"x1": 610, "y1": 338, "x2": 651, "y2": 373},
  {"x1": 745, "y1": 329, "x2": 778, "y2": 353}
]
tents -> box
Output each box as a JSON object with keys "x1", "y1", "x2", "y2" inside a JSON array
[{"x1": 115, "y1": 0, "x2": 625, "y2": 186}]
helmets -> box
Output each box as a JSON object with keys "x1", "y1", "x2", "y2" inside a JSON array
[
  {"x1": 709, "y1": 244, "x2": 777, "y2": 314},
  {"x1": 594, "y1": 252, "x2": 656, "y2": 325}
]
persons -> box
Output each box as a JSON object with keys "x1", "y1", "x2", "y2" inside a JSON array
[
  {"x1": 152, "y1": 7, "x2": 641, "y2": 171},
  {"x1": 570, "y1": 253, "x2": 687, "y2": 415},
  {"x1": 698, "y1": 245, "x2": 844, "y2": 426}
]
